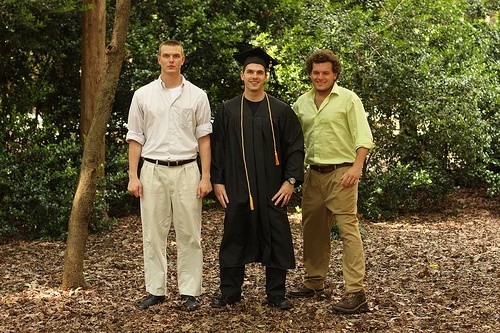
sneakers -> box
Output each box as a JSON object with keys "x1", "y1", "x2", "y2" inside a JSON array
[
  {"x1": 288, "y1": 284, "x2": 324, "y2": 298},
  {"x1": 333, "y1": 291, "x2": 367, "y2": 312}
]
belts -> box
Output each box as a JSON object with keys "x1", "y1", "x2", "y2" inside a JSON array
[
  {"x1": 310, "y1": 163, "x2": 353, "y2": 174},
  {"x1": 146, "y1": 158, "x2": 195, "y2": 166}
]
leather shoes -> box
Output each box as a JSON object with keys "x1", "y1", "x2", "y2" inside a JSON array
[
  {"x1": 182, "y1": 296, "x2": 198, "y2": 311},
  {"x1": 140, "y1": 294, "x2": 165, "y2": 308},
  {"x1": 269, "y1": 297, "x2": 291, "y2": 309},
  {"x1": 212, "y1": 294, "x2": 235, "y2": 308}
]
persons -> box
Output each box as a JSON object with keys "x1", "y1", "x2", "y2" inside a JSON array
[
  {"x1": 292, "y1": 49, "x2": 375, "y2": 311},
  {"x1": 126, "y1": 40, "x2": 212, "y2": 309},
  {"x1": 211, "y1": 47, "x2": 305, "y2": 308}
]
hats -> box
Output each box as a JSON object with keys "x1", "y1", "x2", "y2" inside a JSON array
[{"x1": 232, "y1": 46, "x2": 279, "y2": 71}]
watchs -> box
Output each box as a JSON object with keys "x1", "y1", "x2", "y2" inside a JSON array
[{"x1": 287, "y1": 177, "x2": 296, "y2": 184}]
